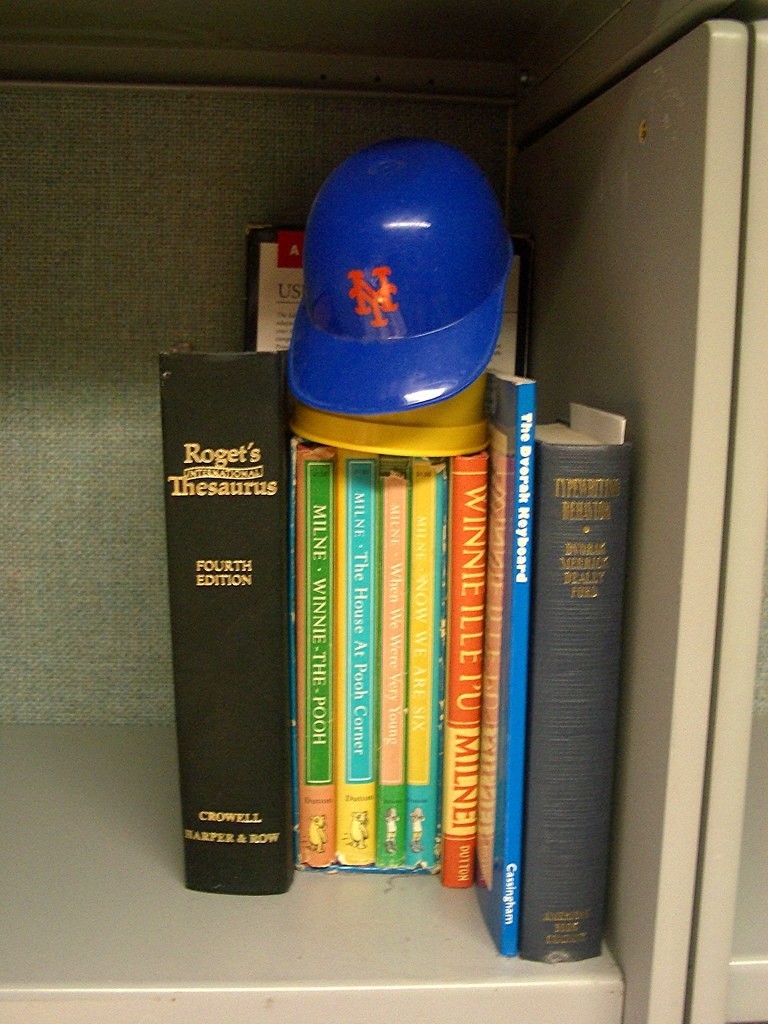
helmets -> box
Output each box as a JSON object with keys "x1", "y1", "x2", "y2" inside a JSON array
[
  {"x1": 288, "y1": 368, "x2": 492, "y2": 457},
  {"x1": 286, "y1": 136, "x2": 513, "y2": 416}
]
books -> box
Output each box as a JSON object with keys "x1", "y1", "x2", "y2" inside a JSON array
[{"x1": 161, "y1": 227, "x2": 633, "y2": 963}]
